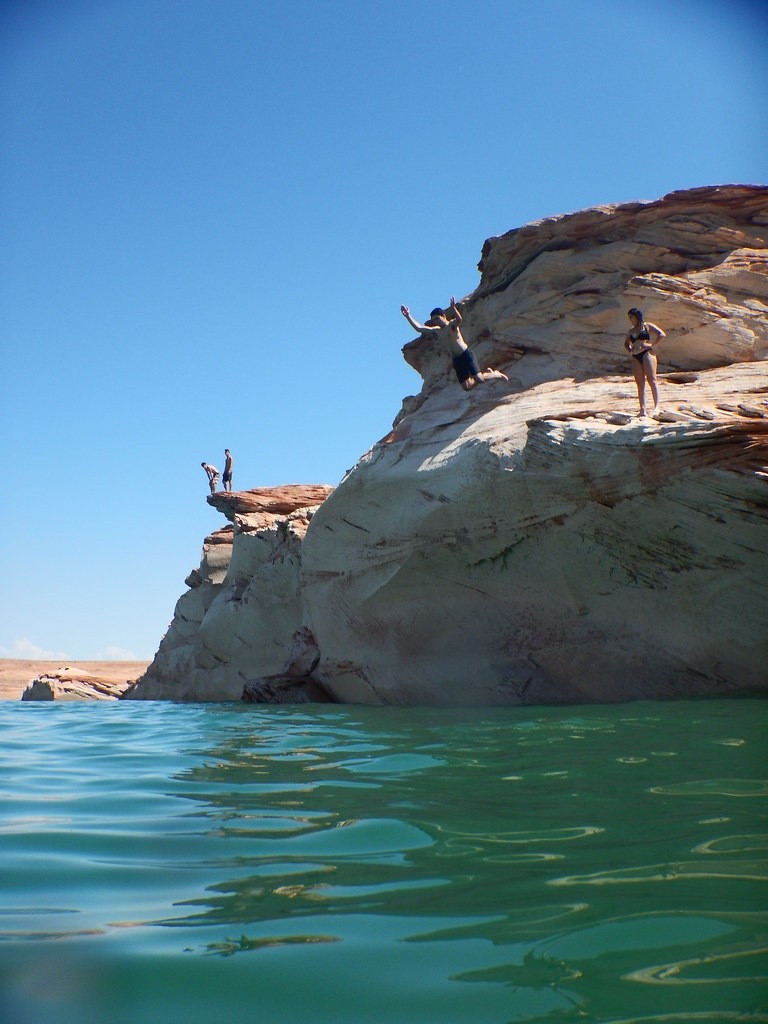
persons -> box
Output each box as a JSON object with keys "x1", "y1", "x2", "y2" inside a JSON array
[
  {"x1": 624, "y1": 308, "x2": 666, "y2": 417},
  {"x1": 222, "y1": 448, "x2": 233, "y2": 492},
  {"x1": 201, "y1": 462, "x2": 220, "y2": 494},
  {"x1": 401, "y1": 296, "x2": 508, "y2": 391}
]
